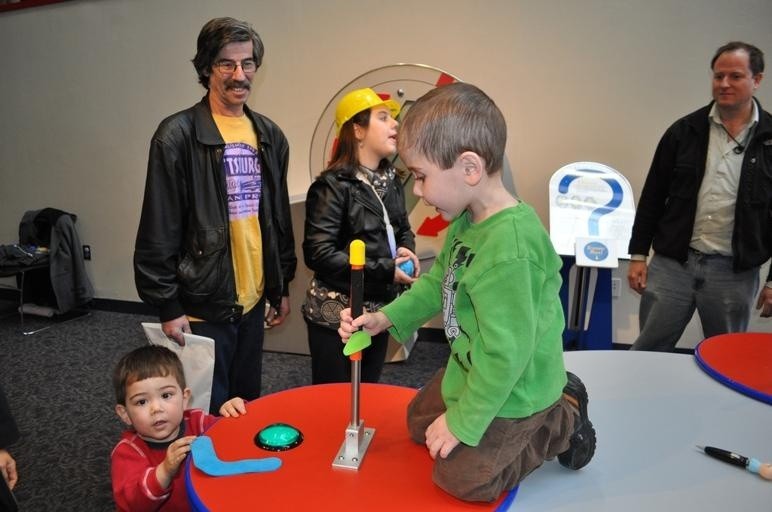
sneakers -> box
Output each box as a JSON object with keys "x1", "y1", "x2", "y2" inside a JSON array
[{"x1": 556, "y1": 370, "x2": 597, "y2": 470}]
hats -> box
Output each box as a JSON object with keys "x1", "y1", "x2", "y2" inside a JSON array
[{"x1": 335, "y1": 88, "x2": 401, "y2": 136}]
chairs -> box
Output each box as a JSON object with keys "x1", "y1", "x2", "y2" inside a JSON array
[{"x1": 0, "y1": 206, "x2": 96, "y2": 336}]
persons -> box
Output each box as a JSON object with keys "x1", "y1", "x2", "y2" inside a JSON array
[
  {"x1": 301, "y1": 84, "x2": 422, "y2": 383},
  {"x1": 109, "y1": 344, "x2": 248, "y2": 510},
  {"x1": 132, "y1": 17, "x2": 297, "y2": 420},
  {"x1": 337, "y1": 78, "x2": 596, "y2": 501},
  {"x1": 626, "y1": 41, "x2": 772, "y2": 357},
  {"x1": 1, "y1": 385, "x2": 27, "y2": 510}
]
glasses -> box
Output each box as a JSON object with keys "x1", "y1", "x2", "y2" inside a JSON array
[{"x1": 213, "y1": 60, "x2": 258, "y2": 74}]
